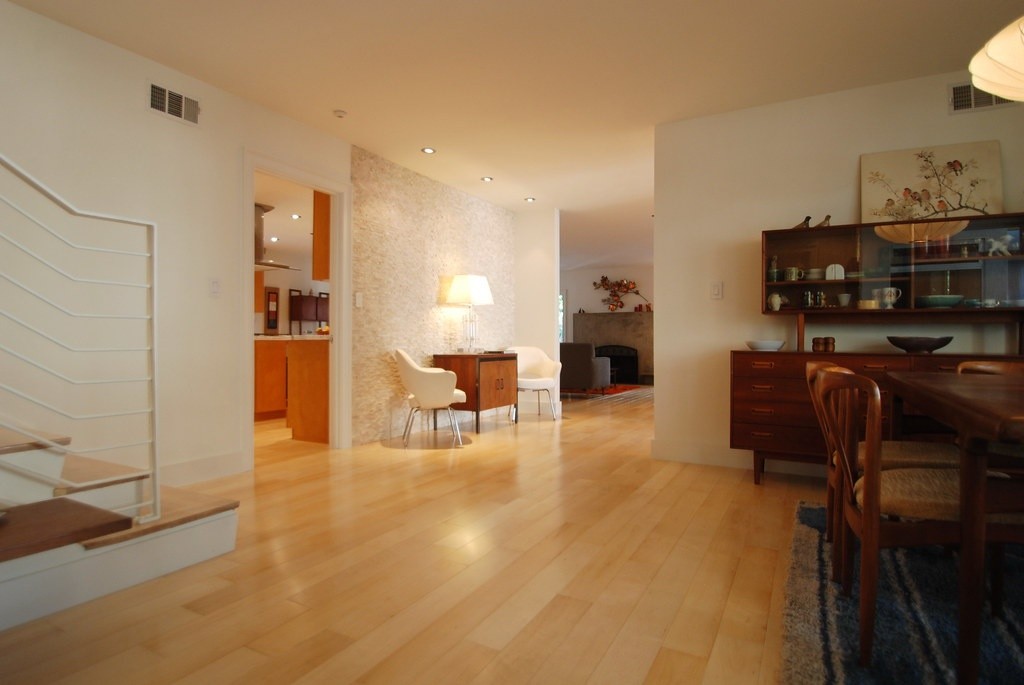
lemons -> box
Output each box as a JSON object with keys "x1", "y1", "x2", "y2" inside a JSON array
[{"x1": 315, "y1": 326, "x2": 329, "y2": 331}]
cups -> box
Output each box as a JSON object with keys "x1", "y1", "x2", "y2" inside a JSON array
[
  {"x1": 837, "y1": 294, "x2": 851, "y2": 307},
  {"x1": 1015, "y1": 299, "x2": 1024, "y2": 307},
  {"x1": 871, "y1": 289, "x2": 881, "y2": 300},
  {"x1": 784, "y1": 267, "x2": 804, "y2": 281}
]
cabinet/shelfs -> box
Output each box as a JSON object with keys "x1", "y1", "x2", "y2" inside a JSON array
[
  {"x1": 729, "y1": 350, "x2": 1024, "y2": 485},
  {"x1": 762, "y1": 212, "x2": 1023, "y2": 323},
  {"x1": 431, "y1": 352, "x2": 519, "y2": 432},
  {"x1": 256, "y1": 340, "x2": 328, "y2": 442}
]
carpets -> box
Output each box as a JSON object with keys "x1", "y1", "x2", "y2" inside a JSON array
[
  {"x1": 561, "y1": 385, "x2": 640, "y2": 396},
  {"x1": 782, "y1": 500, "x2": 1024, "y2": 685}
]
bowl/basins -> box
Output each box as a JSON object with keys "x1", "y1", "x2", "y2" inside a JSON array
[
  {"x1": 919, "y1": 295, "x2": 964, "y2": 309},
  {"x1": 887, "y1": 336, "x2": 953, "y2": 353},
  {"x1": 747, "y1": 341, "x2": 785, "y2": 351},
  {"x1": 962, "y1": 298, "x2": 1000, "y2": 309}
]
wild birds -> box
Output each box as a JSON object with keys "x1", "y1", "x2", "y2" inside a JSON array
[
  {"x1": 792, "y1": 216, "x2": 810, "y2": 228},
  {"x1": 814, "y1": 214, "x2": 831, "y2": 227}
]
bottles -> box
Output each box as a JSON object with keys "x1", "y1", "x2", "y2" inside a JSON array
[
  {"x1": 804, "y1": 268, "x2": 825, "y2": 279},
  {"x1": 771, "y1": 294, "x2": 780, "y2": 312}
]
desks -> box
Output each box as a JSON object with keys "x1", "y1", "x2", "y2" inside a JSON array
[{"x1": 886, "y1": 374, "x2": 1024, "y2": 685}]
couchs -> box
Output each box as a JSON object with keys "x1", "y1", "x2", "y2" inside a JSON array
[{"x1": 558, "y1": 342, "x2": 611, "y2": 399}]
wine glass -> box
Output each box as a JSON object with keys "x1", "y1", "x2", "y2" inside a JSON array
[{"x1": 881, "y1": 287, "x2": 901, "y2": 309}]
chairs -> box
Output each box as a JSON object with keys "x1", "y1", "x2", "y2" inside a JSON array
[
  {"x1": 504, "y1": 346, "x2": 563, "y2": 421},
  {"x1": 804, "y1": 360, "x2": 1024, "y2": 669},
  {"x1": 396, "y1": 349, "x2": 467, "y2": 447}
]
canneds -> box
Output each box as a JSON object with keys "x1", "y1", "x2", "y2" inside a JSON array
[
  {"x1": 961, "y1": 246, "x2": 967, "y2": 258},
  {"x1": 816, "y1": 291, "x2": 823, "y2": 308},
  {"x1": 804, "y1": 290, "x2": 811, "y2": 307}
]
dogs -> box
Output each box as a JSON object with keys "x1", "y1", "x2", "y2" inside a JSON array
[{"x1": 987, "y1": 234, "x2": 1013, "y2": 256}]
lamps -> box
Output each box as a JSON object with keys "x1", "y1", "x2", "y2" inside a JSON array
[
  {"x1": 439, "y1": 273, "x2": 493, "y2": 354},
  {"x1": 969, "y1": 15, "x2": 1023, "y2": 104}
]
